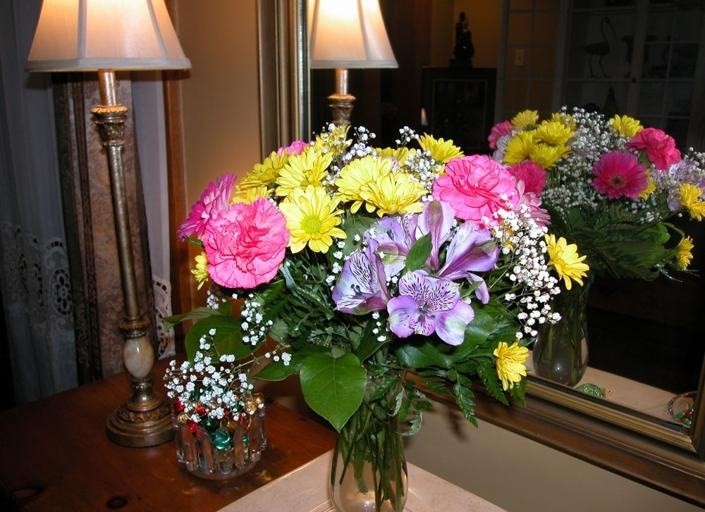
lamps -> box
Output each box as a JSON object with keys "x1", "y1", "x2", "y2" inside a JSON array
[
  {"x1": 306, "y1": 0, "x2": 399, "y2": 169},
  {"x1": 25, "y1": 0, "x2": 192, "y2": 447}
]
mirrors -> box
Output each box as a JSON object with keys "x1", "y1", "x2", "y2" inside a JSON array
[{"x1": 257, "y1": 1, "x2": 704, "y2": 507}]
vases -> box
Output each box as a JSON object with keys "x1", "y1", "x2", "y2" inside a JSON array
[
  {"x1": 170, "y1": 407, "x2": 264, "y2": 481},
  {"x1": 530, "y1": 269, "x2": 591, "y2": 387},
  {"x1": 329, "y1": 377, "x2": 407, "y2": 512}
]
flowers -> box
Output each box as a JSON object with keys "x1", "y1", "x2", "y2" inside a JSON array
[
  {"x1": 487, "y1": 106, "x2": 704, "y2": 387},
  {"x1": 162, "y1": 120, "x2": 589, "y2": 512},
  {"x1": 162, "y1": 329, "x2": 264, "y2": 471}
]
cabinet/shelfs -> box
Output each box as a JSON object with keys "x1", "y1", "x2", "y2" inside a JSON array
[{"x1": 552, "y1": 0, "x2": 705, "y2": 153}]
